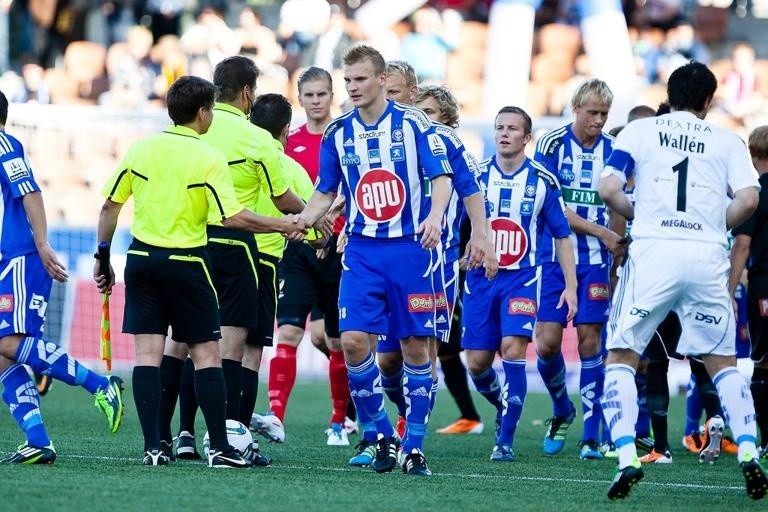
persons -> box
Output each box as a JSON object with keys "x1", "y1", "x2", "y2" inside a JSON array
[{"x1": 0, "y1": 0, "x2": 767, "y2": 503}]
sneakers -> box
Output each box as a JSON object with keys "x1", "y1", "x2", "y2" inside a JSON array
[
  {"x1": 543, "y1": 401, "x2": 576, "y2": 454},
  {"x1": 95, "y1": 375, "x2": 123, "y2": 432},
  {"x1": 250, "y1": 411, "x2": 284, "y2": 443},
  {"x1": 325, "y1": 416, "x2": 358, "y2": 445},
  {"x1": 490, "y1": 413, "x2": 514, "y2": 462},
  {"x1": 578, "y1": 438, "x2": 672, "y2": 464},
  {"x1": 682, "y1": 415, "x2": 768, "y2": 465},
  {"x1": 740, "y1": 450, "x2": 768, "y2": 499},
  {"x1": 349, "y1": 415, "x2": 431, "y2": 475},
  {"x1": 607, "y1": 456, "x2": 644, "y2": 500},
  {"x1": 1, "y1": 442, "x2": 55, "y2": 463},
  {"x1": 142, "y1": 431, "x2": 272, "y2": 467},
  {"x1": 436, "y1": 418, "x2": 484, "y2": 434}
]
